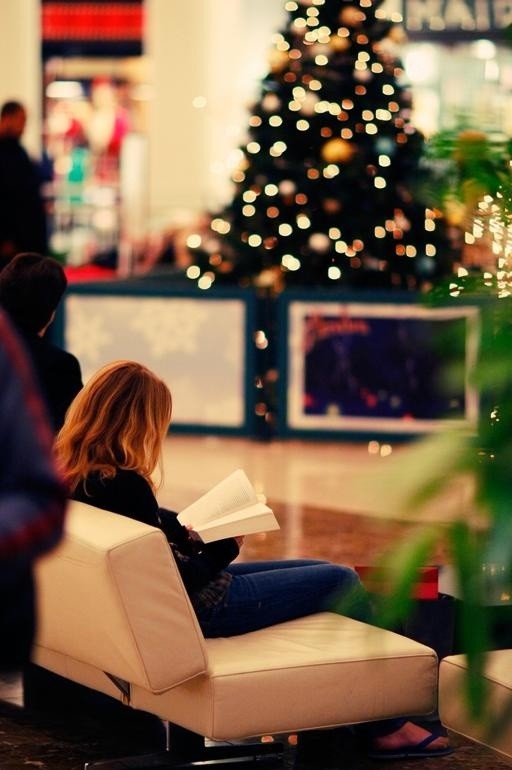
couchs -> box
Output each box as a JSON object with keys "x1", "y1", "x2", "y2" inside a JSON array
[{"x1": 30, "y1": 500, "x2": 440, "y2": 768}]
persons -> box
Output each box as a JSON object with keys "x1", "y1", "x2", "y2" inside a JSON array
[
  {"x1": 0, "y1": 102, "x2": 54, "y2": 253},
  {"x1": 0, "y1": 253, "x2": 83, "y2": 432},
  {"x1": 0, "y1": 310, "x2": 69, "y2": 705},
  {"x1": 52, "y1": 359, "x2": 448, "y2": 750}
]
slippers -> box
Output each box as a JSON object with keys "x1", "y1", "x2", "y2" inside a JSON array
[{"x1": 365, "y1": 732, "x2": 456, "y2": 759}]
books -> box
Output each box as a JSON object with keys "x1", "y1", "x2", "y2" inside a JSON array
[{"x1": 176, "y1": 469, "x2": 280, "y2": 545}]
